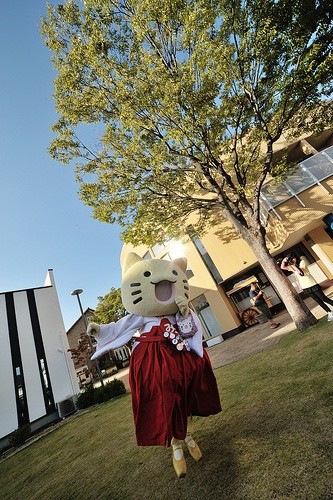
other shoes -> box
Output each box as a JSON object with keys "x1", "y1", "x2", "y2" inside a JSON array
[
  {"x1": 186, "y1": 438, "x2": 203, "y2": 463},
  {"x1": 172, "y1": 447, "x2": 187, "y2": 478},
  {"x1": 271, "y1": 322, "x2": 280, "y2": 328},
  {"x1": 328, "y1": 311, "x2": 333, "y2": 322}
]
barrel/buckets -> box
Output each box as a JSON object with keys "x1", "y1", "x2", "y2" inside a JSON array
[{"x1": 256, "y1": 313, "x2": 268, "y2": 324}]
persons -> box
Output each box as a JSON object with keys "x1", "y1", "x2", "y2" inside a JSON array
[
  {"x1": 249, "y1": 282, "x2": 281, "y2": 329},
  {"x1": 86, "y1": 252, "x2": 224, "y2": 478},
  {"x1": 281, "y1": 252, "x2": 333, "y2": 322}
]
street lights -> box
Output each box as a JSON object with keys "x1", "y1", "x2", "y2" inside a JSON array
[{"x1": 71, "y1": 289, "x2": 104, "y2": 386}]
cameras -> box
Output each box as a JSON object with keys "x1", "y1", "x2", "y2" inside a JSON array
[{"x1": 286, "y1": 256, "x2": 293, "y2": 265}]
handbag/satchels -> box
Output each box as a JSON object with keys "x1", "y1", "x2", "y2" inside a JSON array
[{"x1": 250, "y1": 298, "x2": 262, "y2": 307}]
